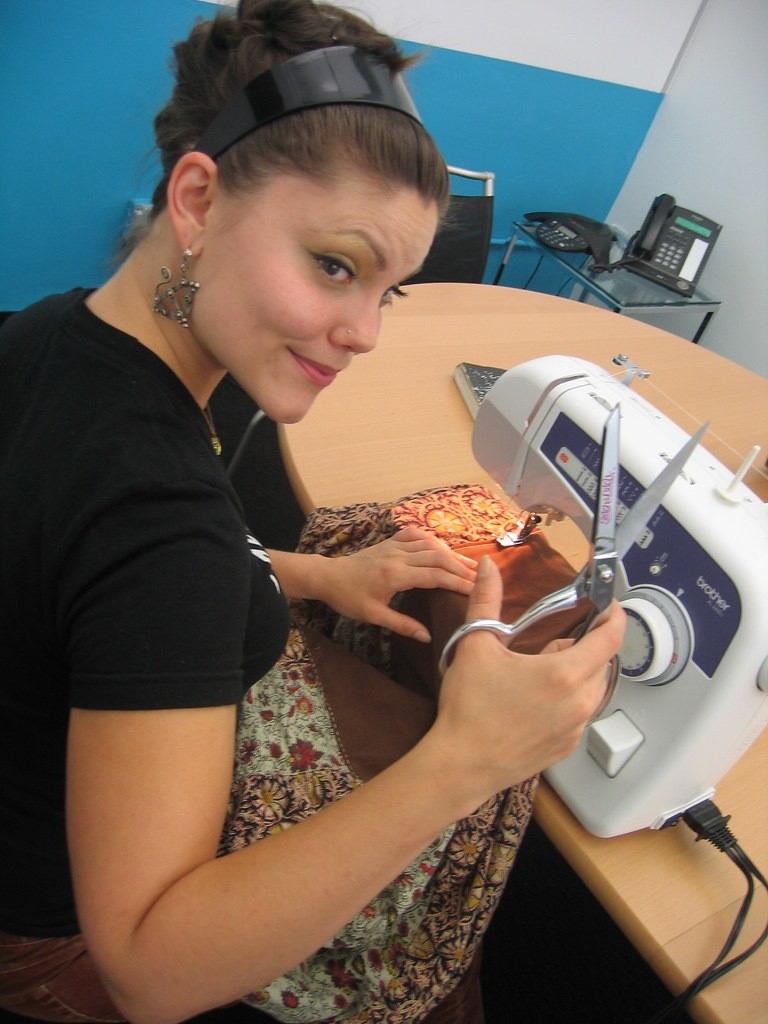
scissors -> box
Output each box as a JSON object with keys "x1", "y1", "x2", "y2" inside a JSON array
[{"x1": 438, "y1": 403, "x2": 712, "y2": 720}]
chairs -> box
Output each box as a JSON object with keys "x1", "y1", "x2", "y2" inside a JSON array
[{"x1": 397, "y1": 164, "x2": 494, "y2": 287}]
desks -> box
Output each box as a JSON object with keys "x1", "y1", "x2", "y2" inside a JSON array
[
  {"x1": 646, "y1": 882, "x2": 768, "y2": 1024},
  {"x1": 277, "y1": 282, "x2": 768, "y2": 965},
  {"x1": 491, "y1": 221, "x2": 723, "y2": 345}
]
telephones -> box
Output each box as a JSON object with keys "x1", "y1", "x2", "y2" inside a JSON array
[{"x1": 621, "y1": 193, "x2": 724, "y2": 299}]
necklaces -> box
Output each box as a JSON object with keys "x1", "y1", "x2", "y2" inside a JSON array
[{"x1": 201, "y1": 402, "x2": 222, "y2": 456}]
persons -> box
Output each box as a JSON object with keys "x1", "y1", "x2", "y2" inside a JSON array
[{"x1": 0, "y1": 1, "x2": 629, "y2": 1024}]
